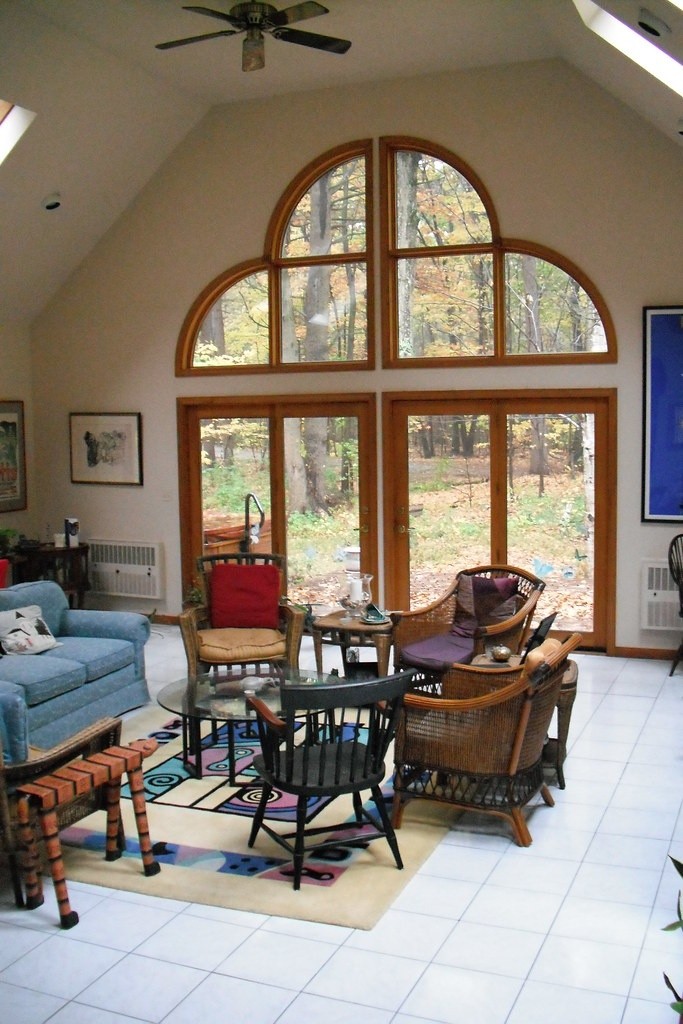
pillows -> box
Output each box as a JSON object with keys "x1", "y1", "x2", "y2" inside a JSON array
[
  {"x1": 451, "y1": 572, "x2": 521, "y2": 637},
  {"x1": 0, "y1": 605, "x2": 64, "y2": 655}
]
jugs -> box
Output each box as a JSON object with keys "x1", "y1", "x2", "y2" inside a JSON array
[{"x1": 490, "y1": 644, "x2": 512, "y2": 663}]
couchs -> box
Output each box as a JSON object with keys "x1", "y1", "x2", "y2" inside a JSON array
[{"x1": 0, "y1": 580, "x2": 152, "y2": 765}]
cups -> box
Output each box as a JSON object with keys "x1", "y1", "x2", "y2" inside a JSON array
[{"x1": 53, "y1": 534, "x2": 66, "y2": 548}]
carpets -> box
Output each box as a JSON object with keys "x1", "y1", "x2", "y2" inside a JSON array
[{"x1": 0, "y1": 697, "x2": 468, "y2": 931}]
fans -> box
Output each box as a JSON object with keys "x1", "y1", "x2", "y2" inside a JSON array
[{"x1": 154, "y1": 0, "x2": 352, "y2": 55}]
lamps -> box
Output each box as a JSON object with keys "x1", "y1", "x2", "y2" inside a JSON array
[{"x1": 242, "y1": 30, "x2": 265, "y2": 72}]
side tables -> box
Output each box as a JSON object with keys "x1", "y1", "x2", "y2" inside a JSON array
[
  {"x1": 470, "y1": 654, "x2": 580, "y2": 790},
  {"x1": 312, "y1": 610, "x2": 399, "y2": 679}
]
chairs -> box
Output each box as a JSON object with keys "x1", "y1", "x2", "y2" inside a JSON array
[
  {"x1": 668, "y1": 534, "x2": 683, "y2": 677},
  {"x1": 0, "y1": 681, "x2": 125, "y2": 908},
  {"x1": 388, "y1": 564, "x2": 545, "y2": 699},
  {"x1": 178, "y1": 552, "x2": 308, "y2": 712},
  {"x1": 246, "y1": 667, "x2": 417, "y2": 892},
  {"x1": 391, "y1": 633, "x2": 581, "y2": 843}
]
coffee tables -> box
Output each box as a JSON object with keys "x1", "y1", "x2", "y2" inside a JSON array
[{"x1": 157, "y1": 668, "x2": 344, "y2": 787}]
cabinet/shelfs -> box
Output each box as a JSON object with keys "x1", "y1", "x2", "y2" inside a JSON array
[{"x1": 203, "y1": 513, "x2": 271, "y2": 571}]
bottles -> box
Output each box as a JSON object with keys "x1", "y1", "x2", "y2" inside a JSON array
[{"x1": 337, "y1": 571, "x2": 374, "y2": 620}]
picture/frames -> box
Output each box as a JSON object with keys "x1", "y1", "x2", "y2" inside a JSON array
[
  {"x1": 640, "y1": 304, "x2": 683, "y2": 524},
  {"x1": 0, "y1": 400, "x2": 28, "y2": 513},
  {"x1": 68, "y1": 412, "x2": 144, "y2": 486}
]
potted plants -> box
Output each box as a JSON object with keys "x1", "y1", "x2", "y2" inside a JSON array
[
  {"x1": 11, "y1": 542, "x2": 92, "y2": 610},
  {"x1": 0, "y1": 527, "x2": 19, "y2": 555}
]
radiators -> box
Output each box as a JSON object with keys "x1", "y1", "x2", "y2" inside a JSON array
[
  {"x1": 640, "y1": 561, "x2": 683, "y2": 631},
  {"x1": 85, "y1": 538, "x2": 164, "y2": 601}
]
